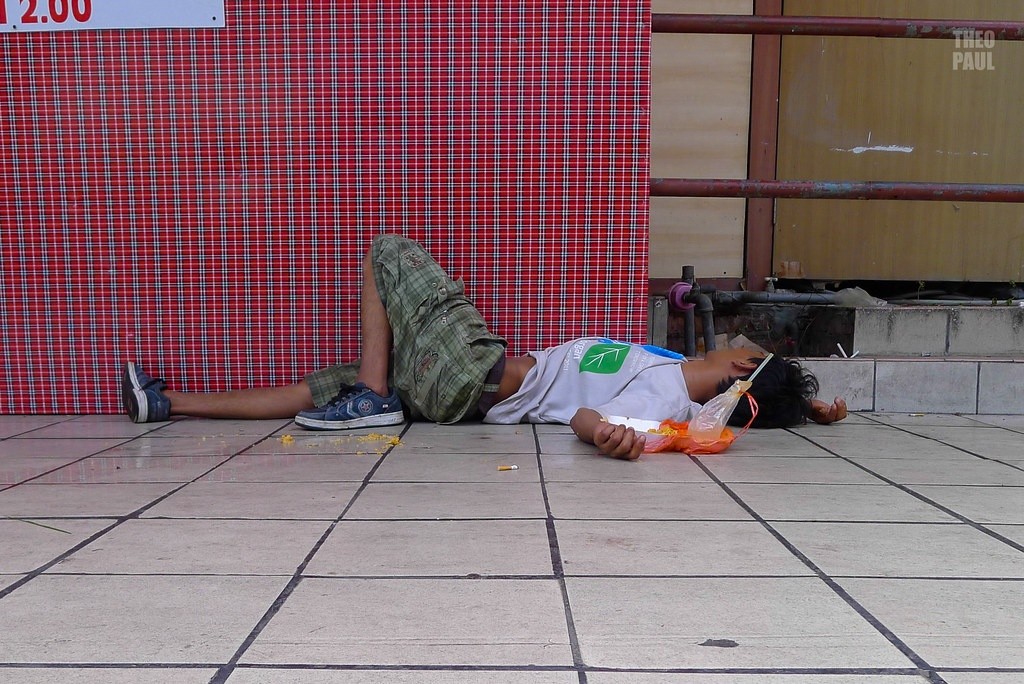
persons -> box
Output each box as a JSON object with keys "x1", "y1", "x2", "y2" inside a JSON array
[{"x1": 121, "y1": 233, "x2": 848, "y2": 461}]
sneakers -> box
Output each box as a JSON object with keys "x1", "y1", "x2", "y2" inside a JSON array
[
  {"x1": 294, "y1": 382, "x2": 405, "y2": 430},
  {"x1": 121, "y1": 362, "x2": 172, "y2": 424}
]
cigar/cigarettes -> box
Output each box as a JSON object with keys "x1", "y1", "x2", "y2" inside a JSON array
[
  {"x1": 908, "y1": 413, "x2": 924, "y2": 416},
  {"x1": 837, "y1": 343, "x2": 847, "y2": 357},
  {"x1": 850, "y1": 351, "x2": 859, "y2": 359},
  {"x1": 498, "y1": 465, "x2": 519, "y2": 471}
]
